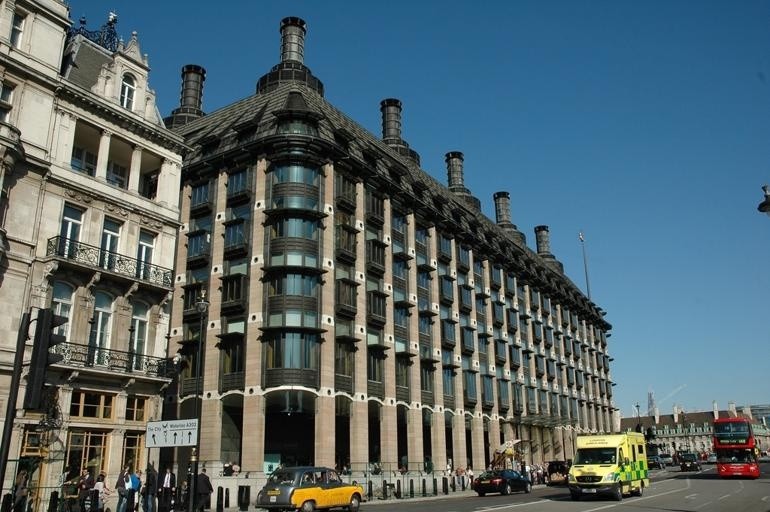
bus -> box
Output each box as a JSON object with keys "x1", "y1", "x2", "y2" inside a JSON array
[
  {"x1": 646, "y1": 441, "x2": 659, "y2": 470},
  {"x1": 711, "y1": 417, "x2": 762, "y2": 480}
]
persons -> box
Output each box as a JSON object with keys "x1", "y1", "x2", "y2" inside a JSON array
[
  {"x1": 11, "y1": 463, "x2": 214, "y2": 512},
  {"x1": 528, "y1": 462, "x2": 547, "y2": 485},
  {"x1": 487, "y1": 464, "x2": 493, "y2": 470},
  {"x1": 370, "y1": 462, "x2": 384, "y2": 475},
  {"x1": 456, "y1": 464, "x2": 474, "y2": 491}
]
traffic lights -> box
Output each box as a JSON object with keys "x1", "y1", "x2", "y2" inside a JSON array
[{"x1": 23, "y1": 308, "x2": 69, "y2": 414}]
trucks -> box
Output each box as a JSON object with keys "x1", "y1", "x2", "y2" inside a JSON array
[{"x1": 569, "y1": 432, "x2": 649, "y2": 500}]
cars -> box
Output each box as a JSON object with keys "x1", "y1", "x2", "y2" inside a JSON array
[
  {"x1": 473, "y1": 469, "x2": 534, "y2": 497},
  {"x1": 679, "y1": 453, "x2": 703, "y2": 472},
  {"x1": 659, "y1": 454, "x2": 673, "y2": 468},
  {"x1": 255, "y1": 466, "x2": 367, "y2": 512}
]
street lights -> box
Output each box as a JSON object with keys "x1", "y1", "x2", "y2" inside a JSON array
[{"x1": 188, "y1": 282, "x2": 211, "y2": 512}]
